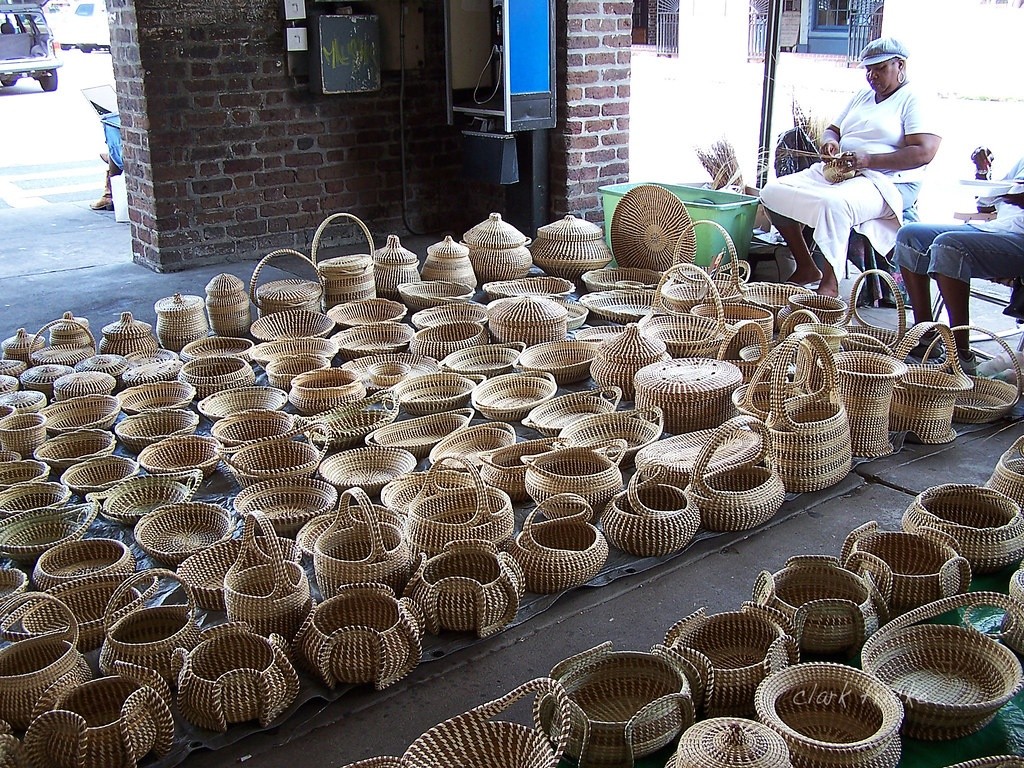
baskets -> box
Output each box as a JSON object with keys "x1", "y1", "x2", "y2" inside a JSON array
[
  {"x1": 983, "y1": 435, "x2": 1024, "y2": 519},
  {"x1": 943, "y1": 755, "x2": 1024, "y2": 768},
  {"x1": 751, "y1": 551, "x2": 893, "y2": 653},
  {"x1": 483, "y1": 184, "x2": 1022, "y2": 595},
  {"x1": 663, "y1": 600, "x2": 800, "y2": 718},
  {"x1": 0, "y1": 212, "x2": 533, "y2": 768},
  {"x1": 402, "y1": 677, "x2": 571, "y2": 768},
  {"x1": 754, "y1": 661, "x2": 904, "y2": 768},
  {"x1": 861, "y1": 591, "x2": 1024, "y2": 740},
  {"x1": 541, "y1": 640, "x2": 703, "y2": 768},
  {"x1": 340, "y1": 756, "x2": 416, "y2": 768},
  {"x1": 840, "y1": 521, "x2": 972, "y2": 619},
  {"x1": 1000, "y1": 559, "x2": 1024, "y2": 655},
  {"x1": 664, "y1": 716, "x2": 793, "y2": 768},
  {"x1": 900, "y1": 482, "x2": 1024, "y2": 576}
]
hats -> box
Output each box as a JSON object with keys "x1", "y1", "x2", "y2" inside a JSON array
[{"x1": 856, "y1": 37, "x2": 910, "y2": 69}]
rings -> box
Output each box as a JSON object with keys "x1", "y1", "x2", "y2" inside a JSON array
[{"x1": 848, "y1": 162, "x2": 852, "y2": 167}]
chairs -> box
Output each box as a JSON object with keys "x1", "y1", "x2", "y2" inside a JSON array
[
  {"x1": 1, "y1": 23, "x2": 15, "y2": 36},
  {"x1": 932, "y1": 272, "x2": 1024, "y2": 361}
]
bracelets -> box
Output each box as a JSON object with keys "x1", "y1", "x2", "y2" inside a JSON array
[{"x1": 976, "y1": 169, "x2": 988, "y2": 174}]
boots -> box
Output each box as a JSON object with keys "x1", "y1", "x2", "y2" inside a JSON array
[
  {"x1": 100, "y1": 153, "x2": 109, "y2": 163},
  {"x1": 90, "y1": 170, "x2": 121, "y2": 211}
]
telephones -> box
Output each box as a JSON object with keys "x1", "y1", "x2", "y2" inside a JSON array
[{"x1": 490, "y1": 4, "x2": 503, "y2": 62}]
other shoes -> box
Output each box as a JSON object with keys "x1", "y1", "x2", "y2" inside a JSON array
[{"x1": 904, "y1": 327, "x2": 976, "y2": 376}]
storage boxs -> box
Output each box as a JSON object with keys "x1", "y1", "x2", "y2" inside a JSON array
[{"x1": 598, "y1": 181, "x2": 761, "y2": 269}]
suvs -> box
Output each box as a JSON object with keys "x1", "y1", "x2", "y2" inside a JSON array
[{"x1": 0, "y1": 0, "x2": 62, "y2": 93}]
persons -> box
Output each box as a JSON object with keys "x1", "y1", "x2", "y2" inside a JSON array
[
  {"x1": 89, "y1": 120, "x2": 122, "y2": 212},
  {"x1": 890, "y1": 155, "x2": 1024, "y2": 374},
  {"x1": 759, "y1": 36, "x2": 942, "y2": 302}
]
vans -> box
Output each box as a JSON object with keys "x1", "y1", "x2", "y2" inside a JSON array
[{"x1": 43, "y1": 0, "x2": 112, "y2": 54}]
